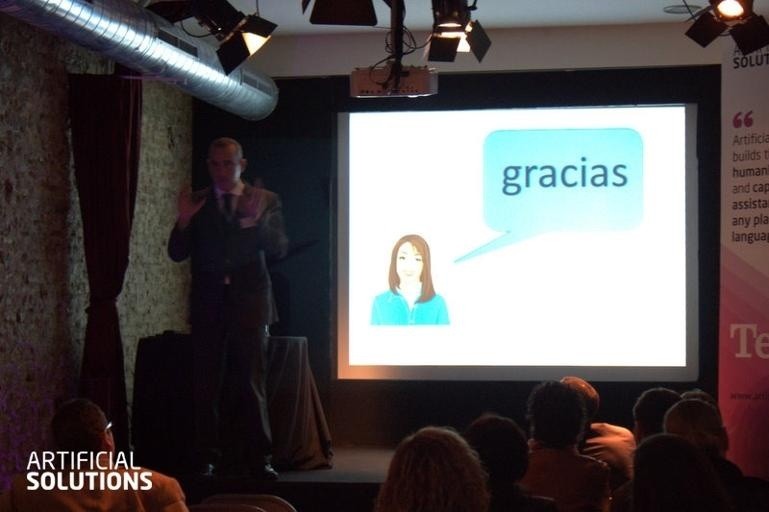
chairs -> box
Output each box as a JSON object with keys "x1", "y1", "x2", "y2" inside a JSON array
[
  {"x1": 206, "y1": 492, "x2": 295, "y2": 511},
  {"x1": 188, "y1": 505, "x2": 265, "y2": 511}
]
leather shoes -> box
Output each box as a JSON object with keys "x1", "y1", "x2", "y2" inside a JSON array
[
  {"x1": 261, "y1": 463, "x2": 279, "y2": 480},
  {"x1": 199, "y1": 463, "x2": 215, "y2": 476}
]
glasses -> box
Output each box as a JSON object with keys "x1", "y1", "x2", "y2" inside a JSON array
[{"x1": 206, "y1": 160, "x2": 240, "y2": 167}]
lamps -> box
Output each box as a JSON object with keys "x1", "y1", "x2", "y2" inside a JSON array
[
  {"x1": 427, "y1": 0, "x2": 490, "y2": 66},
  {"x1": 167, "y1": 1, "x2": 275, "y2": 75},
  {"x1": 302, "y1": 0, "x2": 405, "y2": 27},
  {"x1": 686, "y1": 0, "x2": 769, "y2": 54}
]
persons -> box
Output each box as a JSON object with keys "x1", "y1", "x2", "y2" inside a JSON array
[
  {"x1": 678, "y1": 389, "x2": 722, "y2": 419},
  {"x1": 371, "y1": 235, "x2": 450, "y2": 326},
  {"x1": 663, "y1": 399, "x2": 768, "y2": 511},
  {"x1": 610, "y1": 387, "x2": 685, "y2": 511},
  {"x1": 516, "y1": 379, "x2": 612, "y2": 511},
  {"x1": 559, "y1": 376, "x2": 637, "y2": 491},
  {"x1": 168, "y1": 135, "x2": 288, "y2": 484},
  {"x1": 629, "y1": 435, "x2": 729, "y2": 510},
  {"x1": 378, "y1": 426, "x2": 492, "y2": 509},
  {"x1": 0, "y1": 400, "x2": 189, "y2": 510},
  {"x1": 464, "y1": 413, "x2": 555, "y2": 511}
]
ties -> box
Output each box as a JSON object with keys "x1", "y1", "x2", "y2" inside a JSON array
[{"x1": 221, "y1": 193, "x2": 233, "y2": 217}]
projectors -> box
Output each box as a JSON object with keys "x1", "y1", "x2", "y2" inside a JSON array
[{"x1": 345, "y1": 60, "x2": 440, "y2": 102}]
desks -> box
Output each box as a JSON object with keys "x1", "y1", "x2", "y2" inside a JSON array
[{"x1": 266, "y1": 335, "x2": 330, "y2": 469}]
